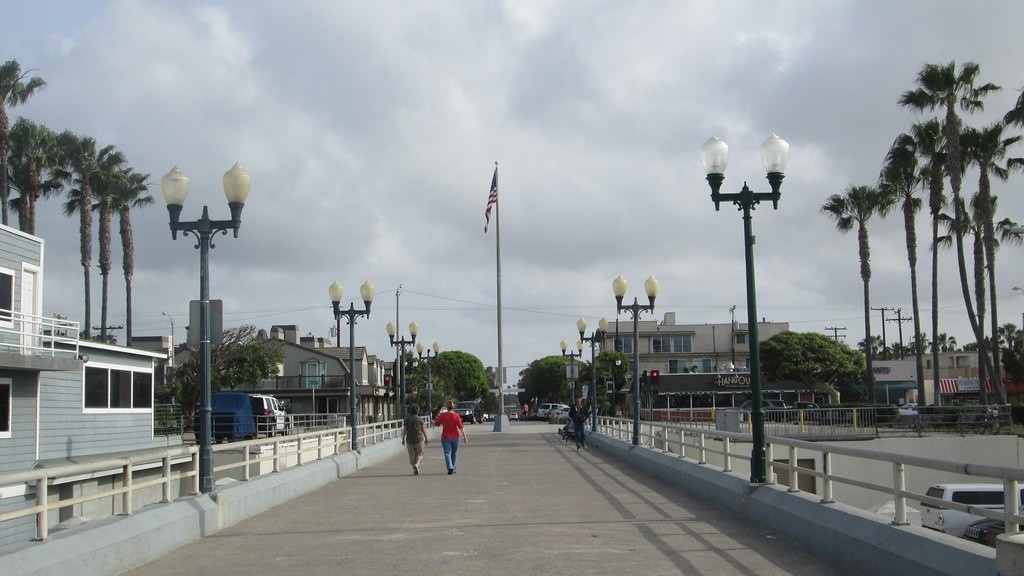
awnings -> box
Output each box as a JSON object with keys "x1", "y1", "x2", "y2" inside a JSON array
[
  {"x1": 939, "y1": 378, "x2": 1007, "y2": 394},
  {"x1": 855, "y1": 381, "x2": 918, "y2": 390}
]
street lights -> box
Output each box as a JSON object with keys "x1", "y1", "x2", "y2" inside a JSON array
[
  {"x1": 162, "y1": 162, "x2": 253, "y2": 494},
  {"x1": 560, "y1": 338, "x2": 583, "y2": 406},
  {"x1": 613, "y1": 275, "x2": 659, "y2": 446},
  {"x1": 700, "y1": 132, "x2": 789, "y2": 482},
  {"x1": 577, "y1": 318, "x2": 608, "y2": 431},
  {"x1": 386, "y1": 321, "x2": 440, "y2": 427},
  {"x1": 329, "y1": 280, "x2": 375, "y2": 451}
]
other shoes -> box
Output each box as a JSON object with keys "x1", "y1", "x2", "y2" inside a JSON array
[
  {"x1": 448, "y1": 470, "x2": 454, "y2": 474},
  {"x1": 577, "y1": 448, "x2": 580, "y2": 451},
  {"x1": 583, "y1": 444, "x2": 587, "y2": 449},
  {"x1": 414, "y1": 465, "x2": 419, "y2": 474}
]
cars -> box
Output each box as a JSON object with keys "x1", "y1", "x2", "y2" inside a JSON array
[
  {"x1": 510, "y1": 412, "x2": 518, "y2": 420},
  {"x1": 551, "y1": 407, "x2": 572, "y2": 424},
  {"x1": 963, "y1": 505, "x2": 1024, "y2": 547}
]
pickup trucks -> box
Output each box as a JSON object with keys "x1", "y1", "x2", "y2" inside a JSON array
[{"x1": 787, "y1": 401, "x2": 819, "y2": 414}]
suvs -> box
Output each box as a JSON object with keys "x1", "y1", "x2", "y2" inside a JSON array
[
  {"x1": 741, "y1": 399, "x2": 786, "y2": 418},
  {"x1": 454, "y1": 401, "x2": 483, "y2": 424},
  {"x1": 246, "y1": 392, "x2": 290, "y2": 437}
]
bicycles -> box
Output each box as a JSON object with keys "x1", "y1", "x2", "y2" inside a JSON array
[{"x1": 976, "y1": 406, "x2": 1000, "y2": 434}]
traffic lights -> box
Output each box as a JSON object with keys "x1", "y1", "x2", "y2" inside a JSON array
[
  {"x1": 651, "y1": 370, "x2": 659, "y2": 384},
  {"x1": 641, "y1": 370, "x2": 647, "y2": 382},
  {"x1": 384, "y1": 374, "x2": 390, "y2": 386}
]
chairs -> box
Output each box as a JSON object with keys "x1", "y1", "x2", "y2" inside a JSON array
[{"x1": 952, "y1": 492, "x2": 971, "y2": 504}]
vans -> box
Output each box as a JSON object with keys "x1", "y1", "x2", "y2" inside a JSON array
[
  {"x1": 536, "y1": 403, "x2": 567, "y2": 422},
  {"x1": 920, "y1": 483, "x2": 1024, "y2": 537}
]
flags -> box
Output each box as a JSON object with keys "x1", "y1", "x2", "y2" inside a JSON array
[{"x1": 484, "y1": 170, "x2": 497, "y2": 234}]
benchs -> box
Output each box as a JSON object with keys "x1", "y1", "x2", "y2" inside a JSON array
[
  {"x1": 558, "y1": 418, "x2": 576, "y2": 443},
  {"x1": 790, "y1": 412, "x2": 805, "y2": 426},
  {"x1": 893, "y1": 414, "x2": 979, "y2": 435},
  {"x1": 806, "y1": 412, "x2": 821, "y2": 426},
  {"x1": 819, "y1": 412, "x2": 837, "y2": 426},
  {"x1": 839, "y1": 412, "x2": 860, "y2": 427}
]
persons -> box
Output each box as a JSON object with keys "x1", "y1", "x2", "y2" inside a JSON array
[
  {"x1": 435, "y1": 400, "x2": 467, "y2": 475},
  {"x1": 402, "y1": 403, "x2": 428, "y2": 475},
  {"x1": 522, "y1": 403, "x2": 528, "y2": 415},
  {"x1": 568, "y1": 397, "x2": 590, "y2": 452}
]
